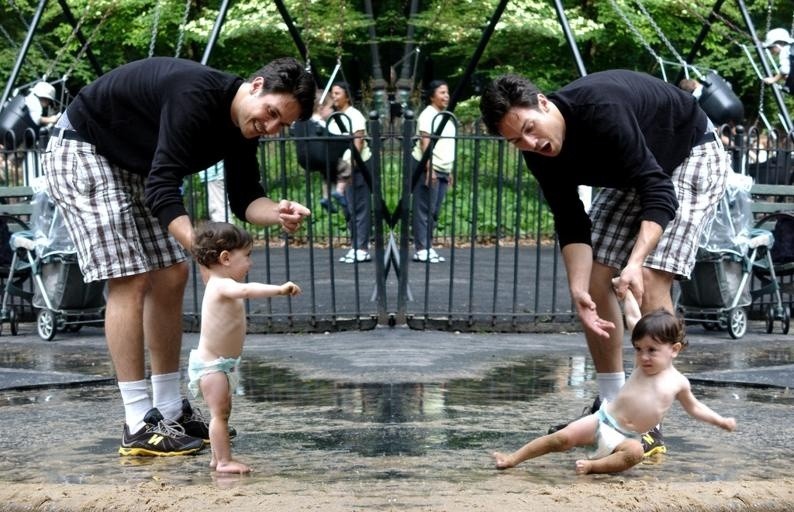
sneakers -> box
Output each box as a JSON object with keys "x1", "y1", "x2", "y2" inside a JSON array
[
  {"x1": 319, "y1": 198, "x2": 337, "y2": 214},
  {"x1": 641, "y1": 427, "x2": 667, "y2": 458},
  {"x1": 548, "y1": 395, "x2": 602, "y2": 436},
  {"x1": 117, "y1": 408, "x2": 205, "y2": 456},
  {"x1": 339, "y1": 249, "x2": 372, "y2": 263},
  {"x1": 332, "y1": 191, "x2": 347, "y2": 207},
  {"x1": 412, "y1": 248, "x2": 445, "y2": 263},
  {"x1": 166, "y1": 399, "x2": 236, "y2": 444}
]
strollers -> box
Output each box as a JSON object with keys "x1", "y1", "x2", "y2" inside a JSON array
[
  {"x1": 672, "y1": 166, "x2": 790, "y2": 342},
  {"x1": 0, "y1": 187, "x2": 113, "y2": 340}
]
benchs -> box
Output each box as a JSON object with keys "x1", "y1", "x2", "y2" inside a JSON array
[
  {"x1": 0, "y1": 186, "x2": 51, "y2": 282},
  {"x1": 734, "y1": 184, "x2": 794, "y2": 319}
]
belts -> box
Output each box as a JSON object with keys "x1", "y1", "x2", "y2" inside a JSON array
[
  {"x1": 50, "y1": 126, "x2": 85, "y2": 142},
  {"x1": 694, "y1": 133, "x2": 715, "y2": 147}
]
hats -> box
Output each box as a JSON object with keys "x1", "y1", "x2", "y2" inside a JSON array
[
  {"x1": 28, "y1": 81, "x2": 57, "y2": 101},
  {"x1": 761, "y1": 27, "x2": 794, "y2": 48}
]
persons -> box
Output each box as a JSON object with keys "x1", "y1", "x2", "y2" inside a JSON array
[
  {"x1": 677, "y1": 78, "x2": 737, "y2": 149},
  {"x1": 197, "y1": 160, "x2": 234, "y2": 226},
  {"x1": 288, "y1": 87, "x2": 349, "y2": 214},
  {"x1": 480, "y1": 68, "x2": 734, "y2": 462},
  {"x1": 491, "y1": 276, "x2": 738, "y2": 477},
  {"x1": 186, "y1": 220, "x2": 303, "y2": 474},
  {"x1": 760, "y1": 26, "x2": 794, "y2": 102},
  {"x1": 41, "y1": 55, "x2": 313, "y2": 457},
  {"x1": 331, "y1": 81, "x2": 374, "y2": 265},
  {"x1": 0, "y1": 80, "x2": 59, "y2": 182},
  {"x1": 410, "y1": 79, "x2": 456, "y2": 263}
]
